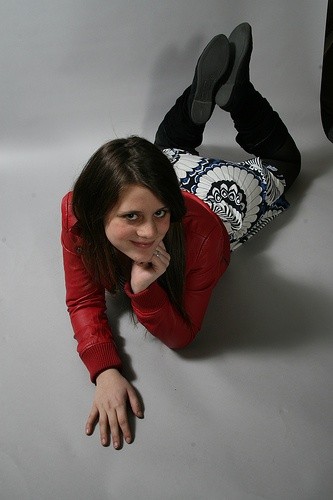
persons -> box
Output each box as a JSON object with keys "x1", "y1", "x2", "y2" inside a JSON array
[{"x1": 61, "y1": 21, "x2": 300, "y2": 449}]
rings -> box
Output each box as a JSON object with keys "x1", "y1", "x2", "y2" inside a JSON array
[{"x1": 156, "y1": 252, "x2": 161, "y2": 257}]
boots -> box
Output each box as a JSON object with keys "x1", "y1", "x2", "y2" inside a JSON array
[
  {"x1": 215, "y1": 22, "x2": 288, "y2": 154},
  {"x1": 157, "y1": 33, "x2": 230, "y2": 149}
]
